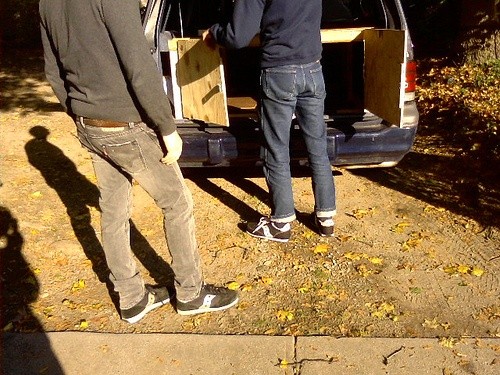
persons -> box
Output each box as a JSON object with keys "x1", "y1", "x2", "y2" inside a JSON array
[
  {"x1": 203, "y1": 0, "x2": 335, "y2": 243},
  {"x1": 39, "y1": 0, "x2": 239, "y2": 323}
]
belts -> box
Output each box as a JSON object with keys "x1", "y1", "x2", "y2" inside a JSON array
[{"x1": 76, "y1": 115, "x2": 142, "y2": 127}]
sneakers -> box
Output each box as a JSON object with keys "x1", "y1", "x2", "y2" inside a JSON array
[
  {"x1": 121, "y1": 286, "x2": 175, "y2": 324},
  {"x1": 177, "y1": 283, "x2": 239, "y2": 316},
  {"x1": 311, "y1": 212, "x2": 335, "y2": 237},
  {"x1": 246, "y1": 216, "x2": 291, "y2": 243}
]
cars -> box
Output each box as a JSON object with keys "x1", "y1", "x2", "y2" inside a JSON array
[{"x1": 140, "y1": 0, "x2": 422, "y2": 170}]
